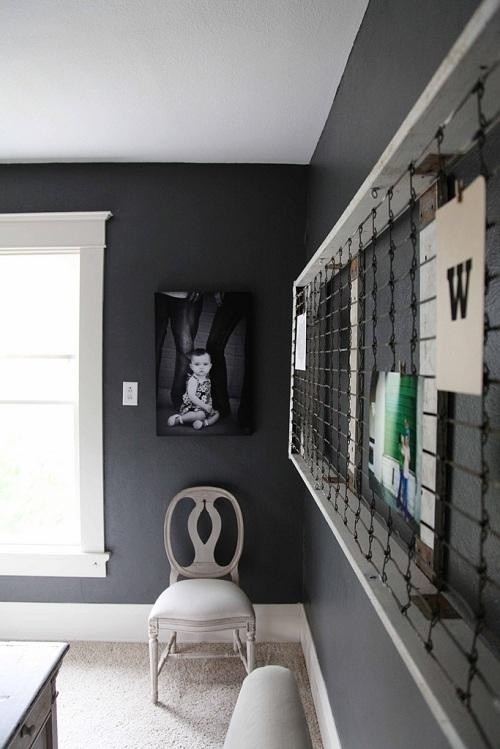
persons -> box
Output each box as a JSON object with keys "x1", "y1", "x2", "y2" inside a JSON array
[
  {"x1": 154, "y1": 291, "x2": 204, "y2": 412},
  {"x1": 396, "y1": 430, "x2": 404, "y2": 507},
  {"x1": 399, "y1": 432, "x2": 411, "y2": 519},
  {"x1": 167, "y1": 347, "x2": 220, "y2": 430},
  {"x1": 205, "y1": 292, "x2": 256, "y2": 437}
]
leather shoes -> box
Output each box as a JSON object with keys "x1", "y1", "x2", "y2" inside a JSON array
[
  {"x1": 193, "y1": 420, "x2": 203, "y2": 429},
  {"x1": 168, "y1": 413, "x2": 183, "y2": 427}
]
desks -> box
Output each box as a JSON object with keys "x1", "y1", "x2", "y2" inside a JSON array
[{"x1": 0, "y1": 640, "x2": 73, "y2": 749}]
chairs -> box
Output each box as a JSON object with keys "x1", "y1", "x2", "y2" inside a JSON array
[{"x1": 146, "y1": 485, "x2": 259, "y2": 704}]
